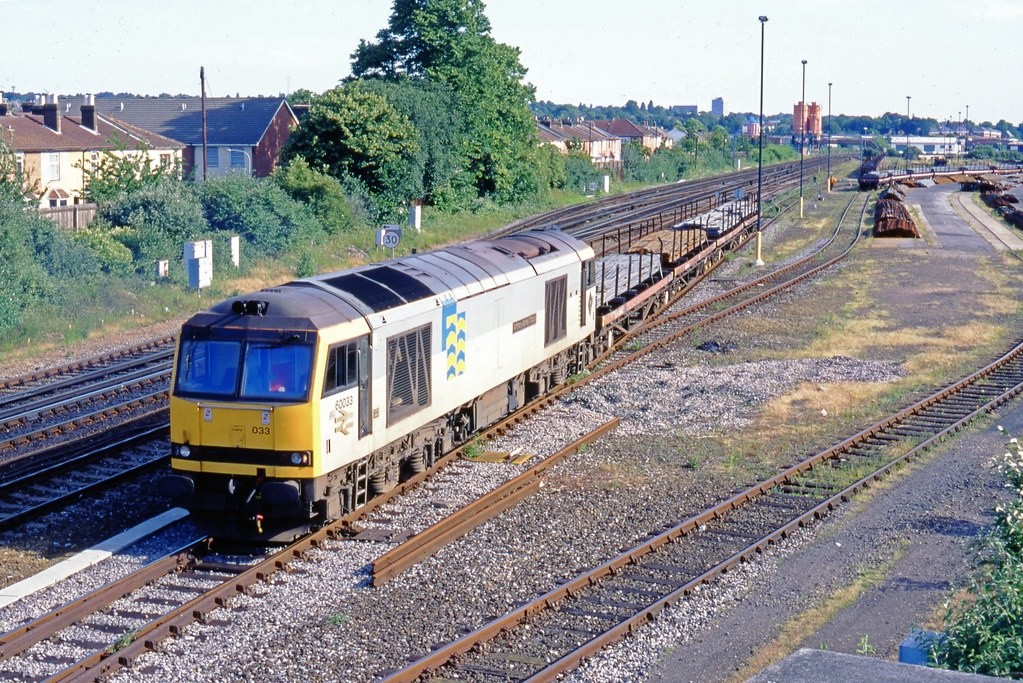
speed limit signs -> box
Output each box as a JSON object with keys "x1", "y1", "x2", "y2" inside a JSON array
[
  {"x1": 382, "y1": 231, "x2": 399, "y2": 249},
  {"x1": 588, "y1": 183, "x2": 598, "y2": 191}
]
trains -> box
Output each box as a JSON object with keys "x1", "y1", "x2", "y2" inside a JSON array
[
  {"x1": 148, "y1": 198, "x2": 758, "y2": 547},
  {"x1": 857, "y1": 164, "x2": 1023, "y2": 191}
]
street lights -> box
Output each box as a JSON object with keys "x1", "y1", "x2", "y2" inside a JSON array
[
  {"x1": 957, "y1": 111, "x2": 962, "y2": 165},
  {"x1": 965, "y1": 105, "x2": 969, "y2": 166},
  {"x1": 825, "y1": 82, "x2": 833, "y2": 194},
  {"x1": 906, "y1": 95, "x2": 911, "y2": 170},
  {"x1": 752, "y1": 14, "x2": 770, "y2": 267},
  {"x1": 948, "y1": 115, "x2": 952, "y2": 159},
  {"x1": 797, "y1": 59, "x2": 809, "y2": 219}
]
